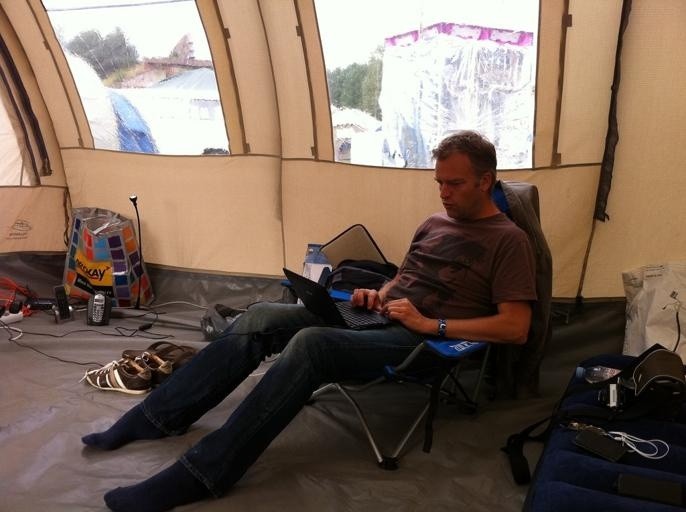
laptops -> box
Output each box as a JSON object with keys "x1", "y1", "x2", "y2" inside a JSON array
[{"x1": 283, "y1": 267, "x2": 396, "y2": 329}]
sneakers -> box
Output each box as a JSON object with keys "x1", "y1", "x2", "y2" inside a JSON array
[
  {"x1": 84, "y1": 357, "x2": 153, "y2": 395},
  {"x1": 132, "y1": 351, "x2": 174, "y2": 388}
]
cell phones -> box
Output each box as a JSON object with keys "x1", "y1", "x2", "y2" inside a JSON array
[{"x1": 573, "y1": 429, "x2": 626, "y2": 463}]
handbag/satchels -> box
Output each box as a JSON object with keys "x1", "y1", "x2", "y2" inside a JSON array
[{"x1": 62, "y1": 206, "x2": 156, "y2": 309}]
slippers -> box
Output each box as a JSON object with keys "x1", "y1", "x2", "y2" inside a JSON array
[{"x1": 121, "y1": 338, "x2": 200, "y2": 370}]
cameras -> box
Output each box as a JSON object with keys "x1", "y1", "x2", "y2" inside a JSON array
[{"x1": 597, "y1": 384, "x2": 625, "y2": 417}]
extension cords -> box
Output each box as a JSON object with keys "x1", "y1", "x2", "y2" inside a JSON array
[{"x1": 0, "y1": 310, "x2": 24, "y2": 327}]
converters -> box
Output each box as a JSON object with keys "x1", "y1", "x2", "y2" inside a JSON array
[{"x1": 9, "y1": 300, "x2": 22, "y2": 313}]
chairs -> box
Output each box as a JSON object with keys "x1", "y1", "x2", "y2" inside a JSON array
[
  {"x1": 304, "y1": 182, "x2": 541, "y2": 471},
  {"x1": 499, "y1": 352, "x2": 686, "y2": 511}
]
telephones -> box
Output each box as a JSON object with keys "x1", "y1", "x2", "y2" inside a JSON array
[
  {"x1": 52, "y1": 285, "x2": 75, "y2": 325},
  {"x1": 87, "y1": 291, "x2": 112, "y2": 326}
]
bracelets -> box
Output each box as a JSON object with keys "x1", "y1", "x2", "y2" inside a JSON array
[{"x1": 438, "y1": 315, "x2": 451, "y2": 343}]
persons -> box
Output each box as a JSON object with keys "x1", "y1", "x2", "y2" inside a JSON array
[{"x1": 80, "y1": 131, "x2": 540, "y2": 512}]
